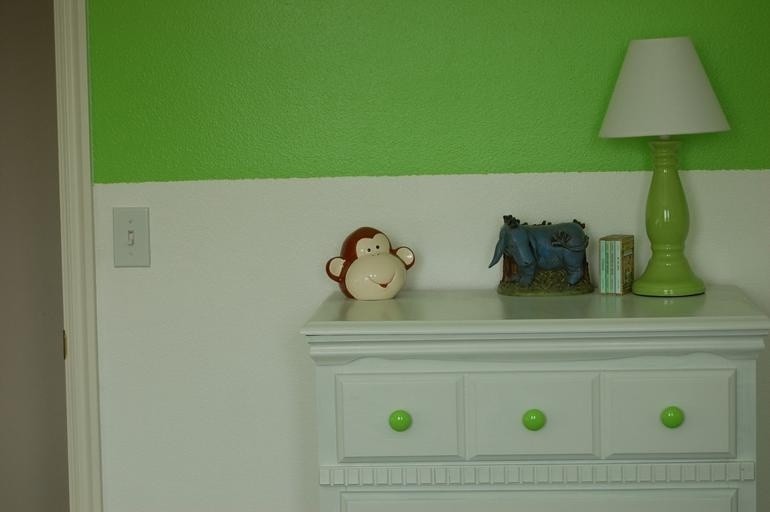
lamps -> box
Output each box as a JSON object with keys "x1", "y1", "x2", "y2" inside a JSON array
[{"x1": 598, "y1": 35, "x2": 730, "y2": 298}]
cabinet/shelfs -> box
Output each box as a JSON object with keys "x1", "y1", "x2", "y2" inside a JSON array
[{"x1": 302, "y1": 286, "x2": 770, "y2": 512}]
